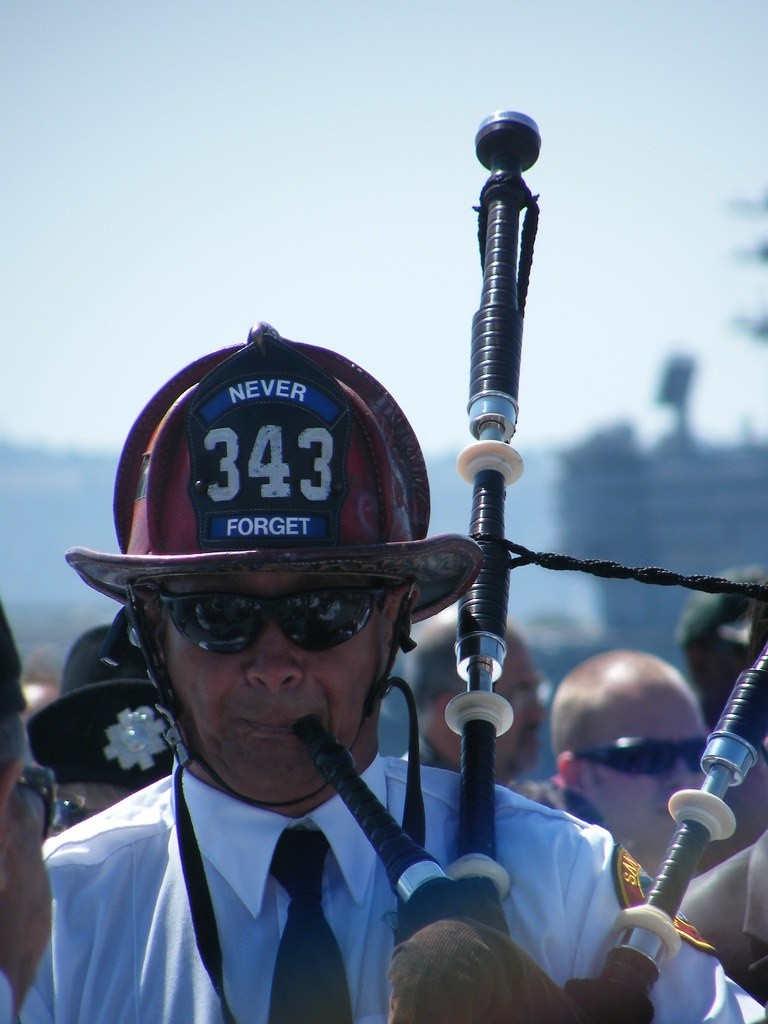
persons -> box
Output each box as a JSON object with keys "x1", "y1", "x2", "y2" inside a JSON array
[{"x1": 0, "y1": 322, "x2": 768, "y2": 1024}]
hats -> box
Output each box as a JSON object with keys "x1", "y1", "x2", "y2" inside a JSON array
[
  {"x1": 0, "y1": 602, "x2": 41, "y2": 715},
  {"x1": 65, "y1": 322, "x2": 485, "y2": 625},
  {"x1": 27, "y1": 624, "x2": 183, "y2": 767}
]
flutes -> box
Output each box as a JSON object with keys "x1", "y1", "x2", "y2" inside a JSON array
[{"x1": 284, "y1": 107, "x2": 766, "y2": 1022}]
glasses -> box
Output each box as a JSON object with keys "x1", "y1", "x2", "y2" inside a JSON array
[
  {"x1": 18, "y1": 768, "x2": 54, "y2": 841},
  {"x1": 451, "y1": 678, "x2": 553, "y2": 714},
  {"x1": 157, "y1": 586, "x2": 385, "y2": 654},
  {"x1": 573, "y1": 736, "x2": 707, "y2": 781}
]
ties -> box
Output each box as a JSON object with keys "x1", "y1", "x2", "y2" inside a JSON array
[{"x1": 268, "y1": 827, "x2": 353, "y2": 1024}]
